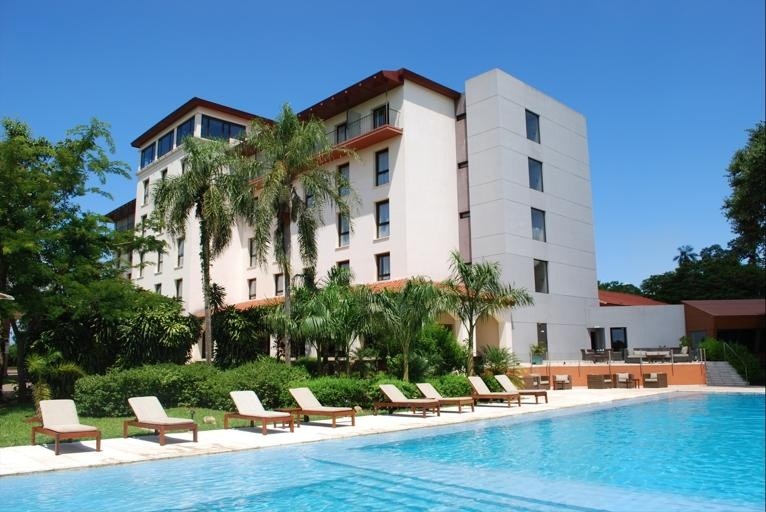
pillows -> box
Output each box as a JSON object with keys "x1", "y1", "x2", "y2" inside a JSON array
[
  {"x1": 627, "y1": 347, "x2": 634, "y2": 355},
  {"x1": 650, "y1": 372, "x2": 657, "y2": 378},
  {"x1": 634, "y1": 351, "x2": 670, "y2": 356},
  {"x1": 680, "y1": 346, "x2": 689, "y2": 354},
  {"x1": 555, "y1": 375, "x2": 568, "y2": 381}
]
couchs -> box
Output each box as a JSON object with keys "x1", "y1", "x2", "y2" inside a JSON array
[{"x1": 633, "y1": 347, "x2": 680, "y2": 363}]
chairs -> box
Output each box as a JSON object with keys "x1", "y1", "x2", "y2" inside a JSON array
[
  {"x1": 587, "y1": 372, "x2": 635, "y2": 388},
  {"x1": 623, "y1": 348, "x2": 643, "y2": 364},
  {"x1": 673, "y1": 346, "x2": 693, "y2": 362},
  {"x1": 552, "y1": 374, "x2": 573, "y2": 389},
  {"x1": 642, "y1": 373, "x2": 668, "y2": 388},
  {"x1": 32, "y1": 394, "x2": 196, "y2": 456},
  {"x1": 580, "y1": 348, "x2": 614, "y2": 363},
  {"x1": 222, "y1": 386, "x2": 358, "y2": 435},
  {"x1": 375, "y1": 373, "x2": 550, "y2": 418}
]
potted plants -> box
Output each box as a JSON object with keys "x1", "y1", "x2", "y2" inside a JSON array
[{"x1": 530, "y1": 341, "x2": 546, "y2": 364}]
